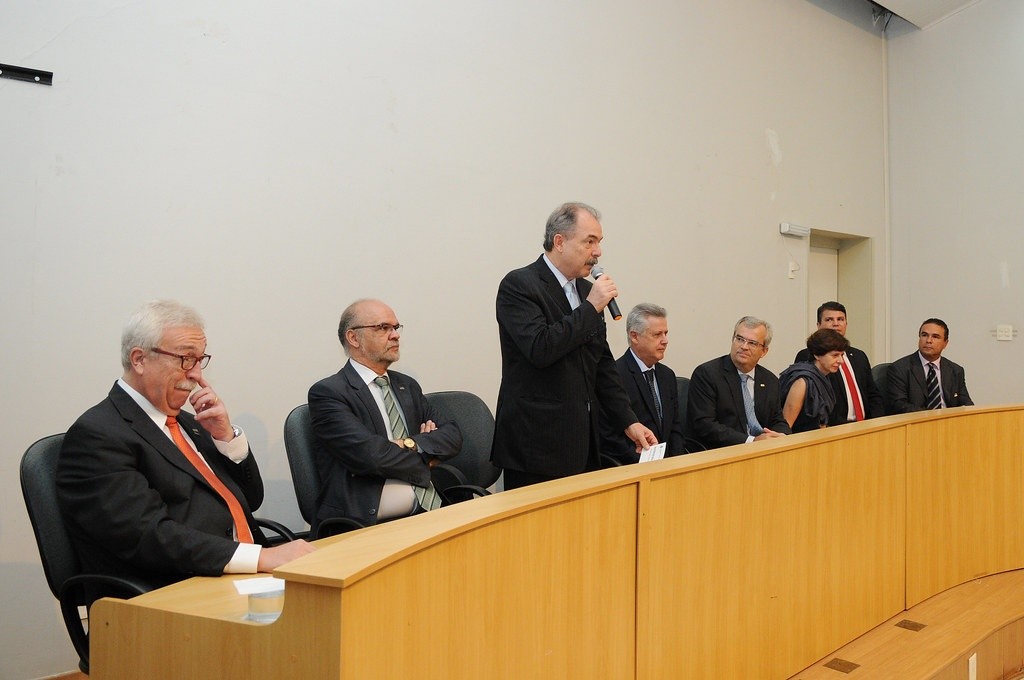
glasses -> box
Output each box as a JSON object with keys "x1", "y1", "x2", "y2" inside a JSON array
[
  {"x1": 149, "y1": 347, "x2": 211, "y2": 370},
  {"x1": 734, "y1": 335, "x2": 765, "y2": 350},
  {"x1": 349, "y1": 323, "x2": 403, "y2": 336}
]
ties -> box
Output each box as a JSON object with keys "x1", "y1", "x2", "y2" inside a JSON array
[
  {"x1": 739, "y1": 373, "x2": 764, "y2": 437},
  {"x1": 564, "y1": 283, "x2": 579, "y2": 311},
  {"x1": 927, "y1": 363, "x2": 942, "y2": 410},
  {"x1": 841, "y1": 359, "x2": 863, "y2": 421},
  {"x1": 374, "y1": 376, "x2": 441, "y2": 511},
  {"x1": 644, "y1": 369, "x2": 661, "y2": 426},
  {"x1": 166, "y1": 415, "x2": 254, "y2": 544}
]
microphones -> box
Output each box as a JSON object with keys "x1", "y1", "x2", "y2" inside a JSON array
[{"x1": 590, "y1": 266, "x2": 622, "y2": 320}]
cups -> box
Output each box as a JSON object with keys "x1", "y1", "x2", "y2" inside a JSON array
[{"x1": 248, "y1": 590, "x2": 285, "y2": 622}]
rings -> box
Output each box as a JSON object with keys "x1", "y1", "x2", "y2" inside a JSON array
[{"x1": 214, "y1": 397, "x2": 217, "y2": 404}]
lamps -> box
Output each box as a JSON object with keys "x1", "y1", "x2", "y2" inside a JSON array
[{"x1": 781, "y1": 223, "x2": 810, "y2": 237}]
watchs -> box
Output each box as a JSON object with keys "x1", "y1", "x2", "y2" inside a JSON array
[
  {"x1": 402, "y1": 437, "x2": 415, "y2": 449},
  {"x1": 232, "y1": 426, "x2": 238, "y2": 437}
]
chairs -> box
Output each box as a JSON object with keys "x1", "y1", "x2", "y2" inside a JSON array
[
  {"x1": 20, "y1": 434, "x2": 297, "y2": 674},
  {"x1": 425, "y1": 391, "x2": 502, "y2": 499},
  {"x1": 284, "y1": 404, "x2": 492, "y2": 542}
]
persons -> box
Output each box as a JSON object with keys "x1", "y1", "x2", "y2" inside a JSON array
[
  {"x1": 687, "y1": 315, "x2": 792, "y2": 452},
  {"x1": 307, "y1": 297, "x2": 462, "y2": 539},
  {"x1": 794, "y1": 300, "x2": 884, "y2": 426},
  {"x1": 489, "y1": 202, "x2": 659, "y2": 491},
  {"x1": 887, "y1": 318, "x2": 974, "y2": 416},
  {"x1": 599, "y1": 303, "x2": 686, "y2": 466},
  {"x1": 779, "y1": 328, "x2": 849, "y2": 434},
  {"x1": 56, "y1": 298, "x2": 317, "y2": 605}
]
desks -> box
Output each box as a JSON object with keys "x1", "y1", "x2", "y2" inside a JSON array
[{"x1": 88, "y1": 405, "x2": 1024, "y2": 680}]
